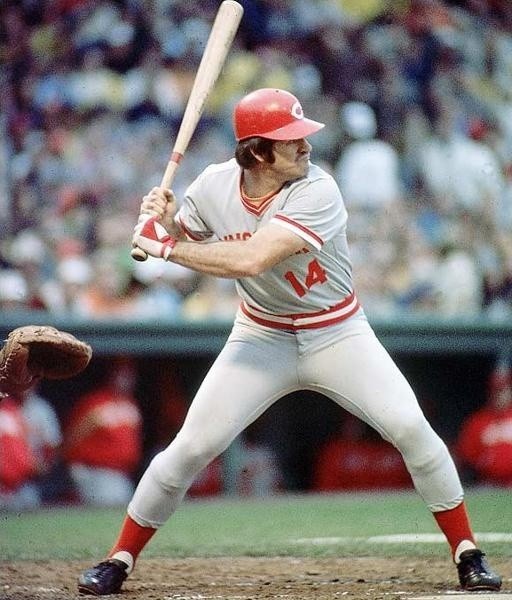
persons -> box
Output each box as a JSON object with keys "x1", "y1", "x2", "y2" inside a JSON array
[{"x1": 77, "y1": 89, "x2": 503, "y2": 595}]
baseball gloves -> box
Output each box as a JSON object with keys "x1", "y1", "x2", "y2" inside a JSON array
[{"x1": 0, "y1": 325, "x2": 92, "y2": 397}]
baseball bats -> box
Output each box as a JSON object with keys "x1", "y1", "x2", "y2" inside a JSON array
[{"x1": 131, "y1": 1, "x2": 244, "y2": 261}]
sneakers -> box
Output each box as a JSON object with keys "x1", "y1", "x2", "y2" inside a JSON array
[
  {"x1": 455, "y1": 548, "x2": 503, "y2": 591},
  {"x1": 78, "y1": 558, "x2": 129, "y2": 595}
]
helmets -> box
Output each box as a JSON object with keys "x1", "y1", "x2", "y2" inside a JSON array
[{"x1": 234, "y1": 88, "x2": 325, "y2": 142}]
